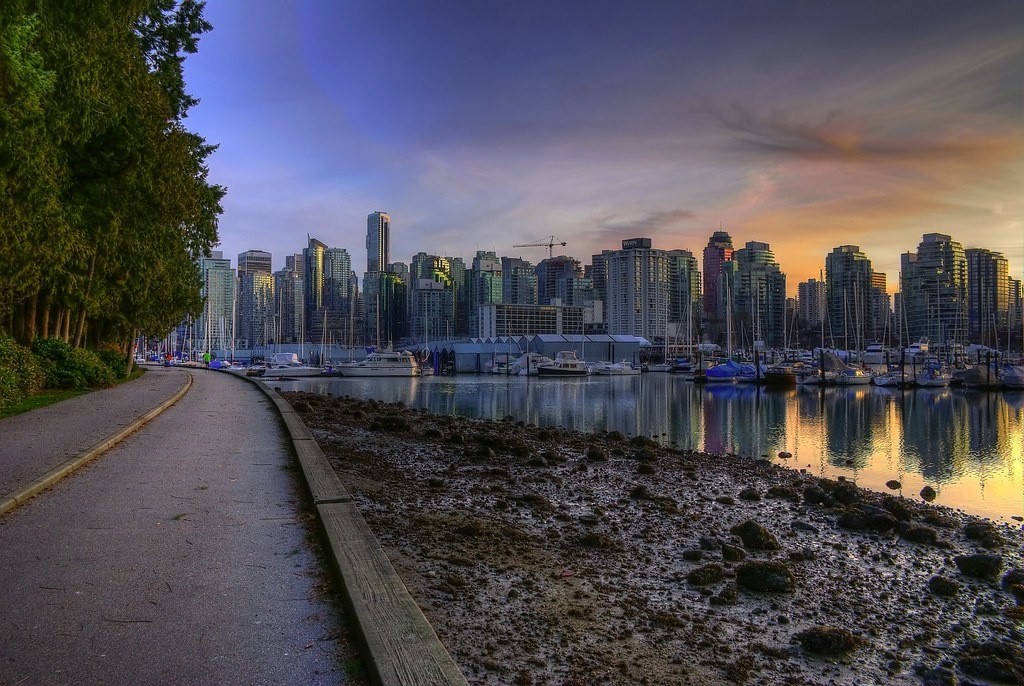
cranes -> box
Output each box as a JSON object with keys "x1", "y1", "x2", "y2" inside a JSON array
[{"x1": 513, "y1": 235, "x2": 569, "y2": 258}]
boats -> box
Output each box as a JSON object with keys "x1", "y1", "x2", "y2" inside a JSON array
[
  {"x1": 261, "y1": 365, "x2": 324, "y2": 376},
  {"x1": 335, "y1": 342, "x2": 418, "y2": 376},
  {"x1": 536, "y1": 350, "x2": 589, "y2": 377},
  {"x1": 597, "y1": 362, "x2": 641, "y2": 375},
  {"x1": 422, "y1": 367, "x2": 434, "y2": 375}
]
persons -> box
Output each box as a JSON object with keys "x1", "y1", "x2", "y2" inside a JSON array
[
  {"x1": 163, "y1": 352, "x2": 172, "y2": 367},
  {"x1": 204, "y1": 350, "x2": 211, "y2": 374}
]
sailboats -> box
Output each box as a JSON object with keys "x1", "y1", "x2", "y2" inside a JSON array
[
  {"x1": 183, "y1": 296, "x2": 392, "y2": 369},
  {"x1": 664, "y1": 269, "x2": 1024, "y2": 388}
]
